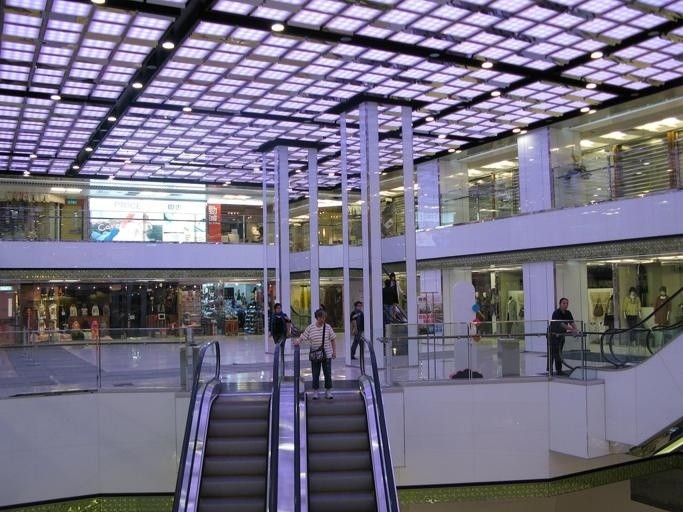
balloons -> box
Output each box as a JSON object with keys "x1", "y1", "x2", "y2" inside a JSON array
[
  {"x1": 471, "y1": 304, "x2": 481, "y2": 312},
  {"x1": 473, "y1": 318, "x2": 481, "y2": 327}
]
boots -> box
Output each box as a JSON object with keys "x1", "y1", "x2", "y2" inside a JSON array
[
  {"x1": 312, "y1": 389, "x2": 320, "y2": 399},
  {"x1": 324, "y1": 388, "x2": 333, "y2": 399}
]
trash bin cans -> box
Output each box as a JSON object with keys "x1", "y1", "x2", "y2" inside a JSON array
[
  {"x1": 498, "y1": 339, "x2": 520, "y2": 378},
  {"x1": 179, "y1": 345, "x2": 200, "y2": 387}
]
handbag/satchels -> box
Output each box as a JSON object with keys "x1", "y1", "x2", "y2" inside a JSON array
[{"x1": 309, "y1": 348, "x2": 327, "y2": 362}]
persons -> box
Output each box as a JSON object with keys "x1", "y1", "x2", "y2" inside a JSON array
[
  {"x1": 350, "y1": 301, "x2": 364, "y2": 359},
  {"x1": 268, "y1": 303, "x2": 291, "y2": 344},
  {"x1": 506, "y1": 297, "x2": 517, "y2": 339},
  {"x1": 623, "y1": 287, "x2": 642, "y2": 345},
  {"x1": 381, "y1": 201, "x2": 395, "y2": 236},
  {"x1": 320, "y1": 304, "x2": 327, "y2": 314},
  {"x1": 606, "y1": 294, "x2": 614, "y2": 329},
  {"x1": 546, "y1": 297, "x2": 583, "y2": 376},
  {"x1": 293, "y1": 309, "x2": 337, "y2": 400},
  {"x1": 654, "y1": 286, "x2": 673, "y2": 324},
  {"x1": 382, "y1": 272, "x2": 400, "y2": 325}
]
